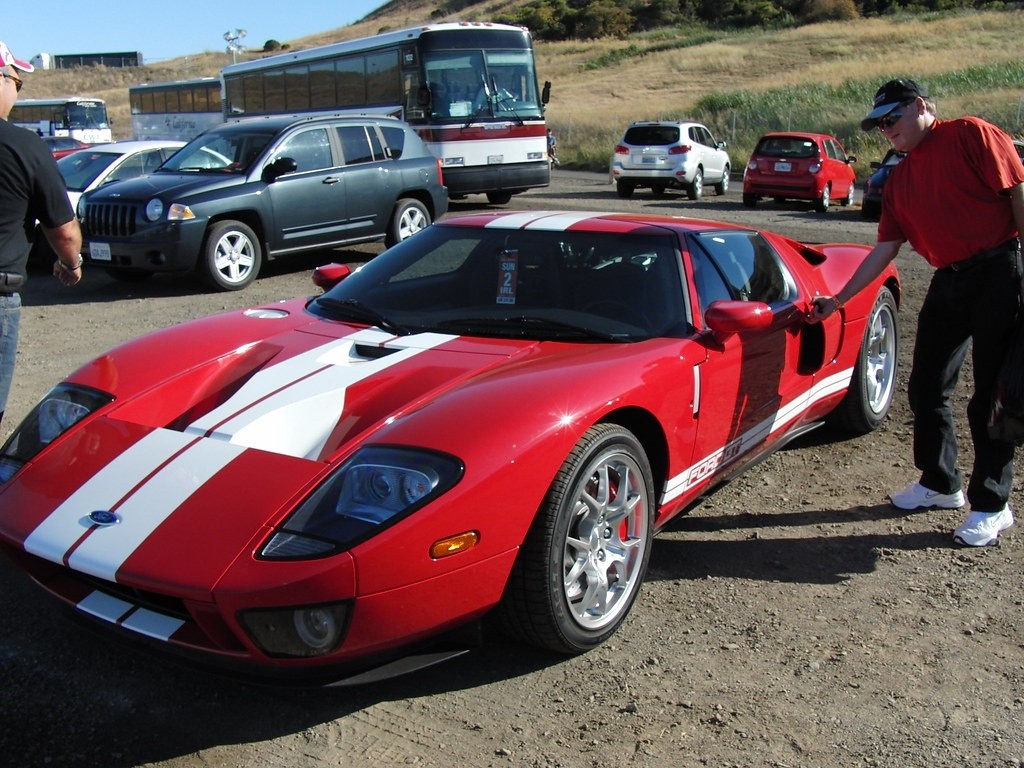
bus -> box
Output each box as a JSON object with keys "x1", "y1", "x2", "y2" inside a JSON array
[
  {"x1": 7, "y1": 96, "x2": 114, "y2": 145},
  {"x1": 219, "y1": 22, "x2": 552, "y2": 206}
]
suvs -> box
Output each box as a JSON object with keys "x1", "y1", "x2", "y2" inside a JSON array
[
  {"x1": 74, "y1": 112, "x2": 448, "y2": 294},
  {"x1": 612, "y1": 120, "x2": 731, "y2": 200}
]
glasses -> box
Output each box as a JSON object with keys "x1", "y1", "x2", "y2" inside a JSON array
[
  {"x1": 0, "y1": 70, "x2": 23, "y2": 92},
  {"x1": 877, "y1": 98, "x2": 917, "y2": 129}
]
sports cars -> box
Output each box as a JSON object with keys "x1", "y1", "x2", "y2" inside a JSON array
[{"x1": 1, "y1": 212, "x2": 901, "y2": 690}]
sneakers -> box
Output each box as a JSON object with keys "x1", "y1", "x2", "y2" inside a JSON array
[
  {"x1": 889, "y1": 482, "x2": 966, "y2": 510},
  {"x1": 953, "y1": 501, "x2": 1015, "y2": 547}
]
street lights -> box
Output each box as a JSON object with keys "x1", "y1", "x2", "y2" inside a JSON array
[{"x1": 223, "y1": 28, "x2": 247, "y2": 65}]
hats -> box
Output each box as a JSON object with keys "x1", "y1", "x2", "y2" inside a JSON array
[
  {"x1": 0, "y1": 40, "x2": 35, "y2": 72},
  {"x1": 861, "y1": 78, "x2": 928, "y2": 132}
]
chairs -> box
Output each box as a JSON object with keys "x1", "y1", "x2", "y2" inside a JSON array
[
  {"x1": 774, "y1": 141, "x2": 810, "y2": 153},
  {"x1": 428, "y1": 81, "x2": 482, "y2": 112},
  {"x1": 472, "y1": 234, "x2": 687, "y2": 324}
]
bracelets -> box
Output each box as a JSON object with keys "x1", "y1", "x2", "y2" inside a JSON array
[{"x1": 832, "y1": 295, "x2": 844, "y2": 308}]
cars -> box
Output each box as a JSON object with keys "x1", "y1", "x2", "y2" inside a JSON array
[
  {"x1": 128, "y1": 76, "x2": 222, "y2": 145},
  {"x1": 862, "y1": 148, "x2": 908, "y2": 220},
  {"x1": 40, "y1": 135, "x2": 90, "y2": 162},
  {"x1": 742, "y1": 133, "x2": 858, "y2": 213},
  {"x1": 35, "y1": 139, "x2": 233, "y2": 227}
]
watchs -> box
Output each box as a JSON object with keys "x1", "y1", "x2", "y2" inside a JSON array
[{"x1": 59, "y1": 253, "x2": 83, "y2": 271}]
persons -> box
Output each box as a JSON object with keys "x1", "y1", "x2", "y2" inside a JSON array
[
  {"x1": 804, "y1": 79, "x2": 1024, "y2": 547},
  {"x1": 475, "y1": 73, "x2": 515, "y2": 112},
  {"x1": 0, "y1": 42, "x2": 83, "y2": 423}
]
